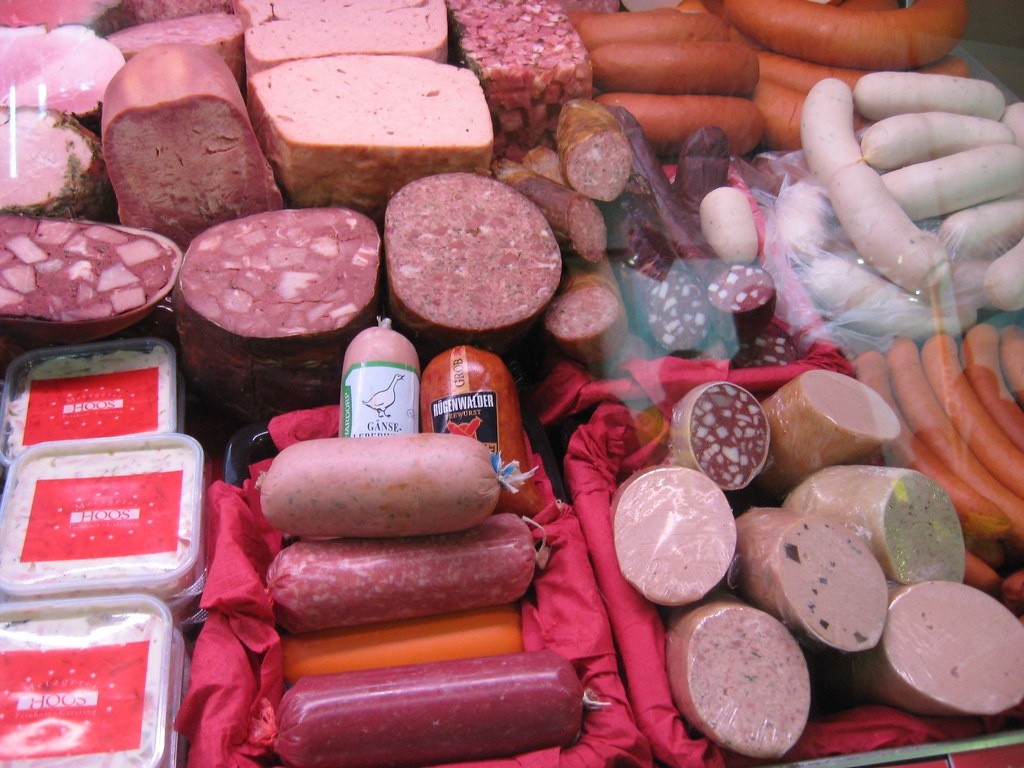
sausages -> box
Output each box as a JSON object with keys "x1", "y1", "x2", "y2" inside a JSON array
[
  {"x1": 254, "y1": 319, "x2": 592, "y2": 768},
  {"x1": 483, "y1": 0, "x2": 1024, "y2": 768}
]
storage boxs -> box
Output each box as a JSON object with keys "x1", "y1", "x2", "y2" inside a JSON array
[{"x1": 1, "y1": 159, "x2": 1022, "y2": 768}]
